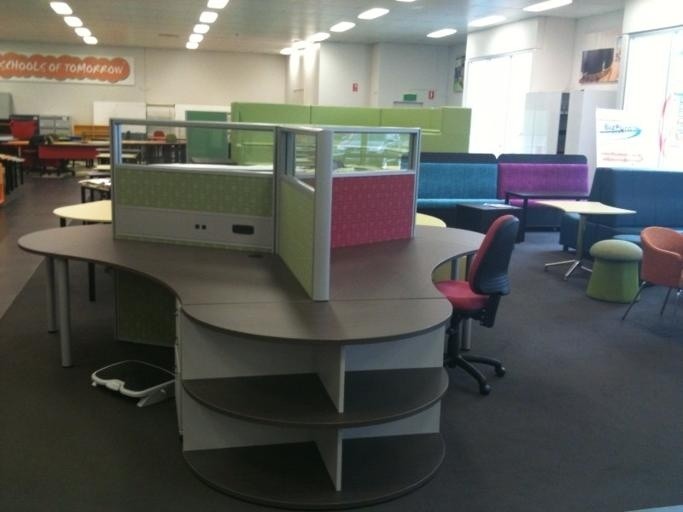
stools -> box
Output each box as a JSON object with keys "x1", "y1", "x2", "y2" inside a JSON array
[
  {"x1": 585, "y1": 239, "x2": 644, "y2": 304},
  {"x1": 455, "y1": 204, "x2": 525, "y2": 243}
]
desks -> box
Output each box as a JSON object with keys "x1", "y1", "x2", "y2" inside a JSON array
[
  {"x1": 532, "y1": 201, "x2": 638, "y2": 281},
  {"x1": 503, "y1": 190, "x2": 590, "y2": 242},
  {"x1": 0, "y1": 134, "x2": 186, "y2": 223},
  {"x1": 15, "y1": 211, "x2": 487, "y2": 435}
]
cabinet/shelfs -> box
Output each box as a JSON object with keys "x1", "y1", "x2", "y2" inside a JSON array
[
  {"x1": 0, "y1": 116, "x2": 110, "y2": 137},
  {"x1": 178, "y1": 298, "x2": 449, "y2": 511}
]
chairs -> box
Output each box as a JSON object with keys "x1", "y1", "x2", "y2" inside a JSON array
[
  {"x1": 153, "y1": 130, "x2": 177, "y2": 142},
  {"x1": 620, "y1": 226, "x2": 683, "y2": 322},
  {"x1": 124, "y1": 131, "x2": 130, "y2": 139},
  {"x1": 434, "y1": 214, "x2": 520, "y2": 396}
]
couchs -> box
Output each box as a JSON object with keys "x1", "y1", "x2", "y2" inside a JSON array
[
  {"x1": 400, "y1": 151, "x2": 498, "y2": 227},
  {"x1": 497, "y1": 154, "x2": 589, "y2": 231},
  {"x1": 559, "y1": 167, "x2": 683, "y2": 253}
]
